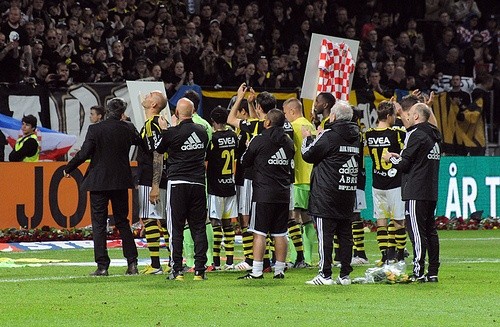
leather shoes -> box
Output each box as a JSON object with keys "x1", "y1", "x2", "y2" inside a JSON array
[
  {"x1": 126, "y1": 263, "x2": 138, "y2": 274},
  {"x1": 89, "y1": 269, "x2": 108, "y2": 276}
]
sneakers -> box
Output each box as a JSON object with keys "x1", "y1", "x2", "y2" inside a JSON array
[
  {"x1": 193, "y1": 270, "x2": 208, "y2": 280},
  {"x1": 222, "y1": 264, "x2": 235, "y2": 272},
  {"x1": 263, "y1": 266, "x2": 272, "y2": 272},
  {"x1": 138, "y1": 264, "x2": 163, "y2": 275},
  {"x1": 238, "y1": 271, "x2": 264, "y2": 280},
  {"x1": 206, "y1": 263, "x2": 221, "y2": 272},
  {"x1": 293, "y1": 260, "x2": 305, "y2": 269},
  {"x1": 350, "y1": 257, "x2": 369, "y2": 267},
  {"x1": 273, "y1": 272, "x2": 284, "y2": 278},
  {"x1": 334, "y1": 275, "x2": 351, "y2": 286},
  {"x1": 235, "y1": 261, "x2": 253, "y2": 271},
  {"x1": 167, "y1": 271, "x2": 184, "y2": 281},
  {"x1": 305, "y1": 275, "x2": 334, "y2": 286}
]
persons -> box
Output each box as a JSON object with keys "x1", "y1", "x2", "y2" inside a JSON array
[
  {"x1": 0, "y1": 0, "x2": 500, "y2": 275},
  {"x1": 9, "y1": 114, "x2": 40, "y2": 162},
  {"x1": 237, "y1": 108, "x2": 295, "y2": 280},
  {"x1": 299, "y1": 99, "x2": 365, "y2": 285},
  {"x1": 381, "y1": 102, "x2": 443, "y2": 284},
  {"x1": 63, "y1": 97, "x2": 146, "y2": 276},
  {"x1": 153, "y1": 98, "x2": 209, "y2": 280}
]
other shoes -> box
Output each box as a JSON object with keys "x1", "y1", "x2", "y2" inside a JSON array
[
  {"x1": 375, "y1": 260, "x2": 385, "y2": 267},
  {"x1": 409, "y1": 274, "x2": 426, "y2": 283},
  {"x1": 425, "y1": 273, "x2": 439, "y2": 282}
]
